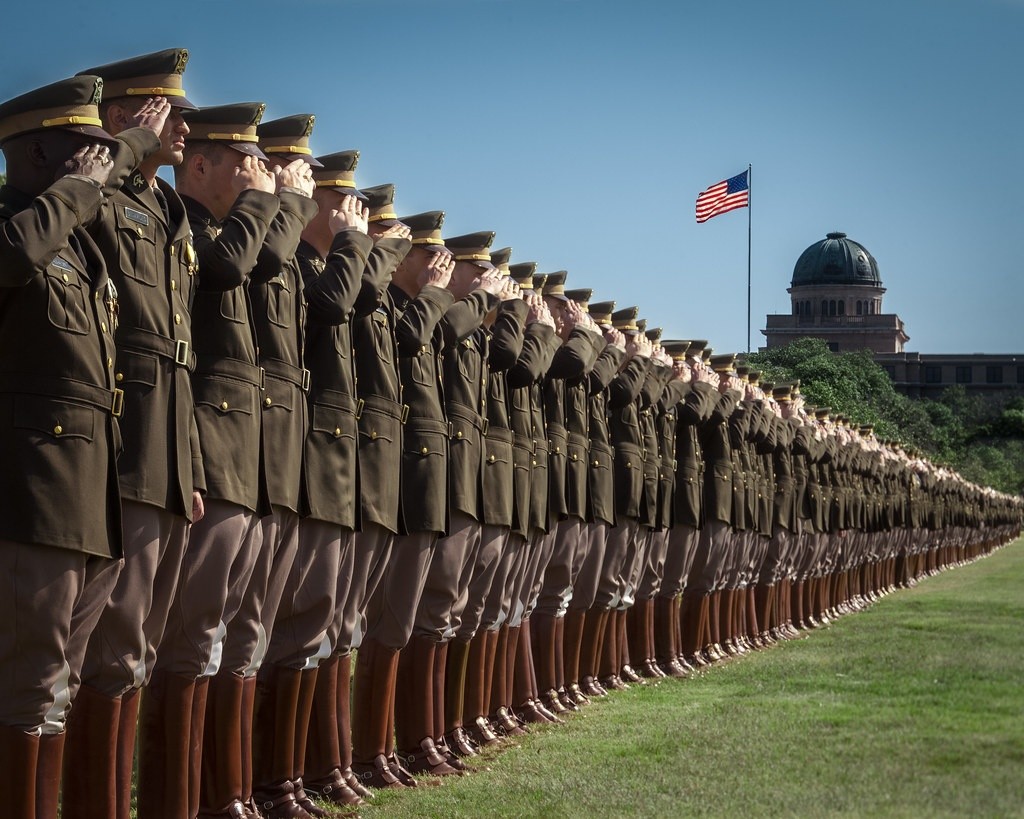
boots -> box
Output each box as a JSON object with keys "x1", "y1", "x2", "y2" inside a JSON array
[{"x1": 0, "y1": 523, "x2": 1023, "y2": 819}]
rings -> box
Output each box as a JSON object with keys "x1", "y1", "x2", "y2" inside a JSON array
[
  {"x1": 440, "y1": 263, "x2": 449, "y2": 269},
  {"x1": 399, "y1": 234, "x2": 404, "y2": 237},
  {"x1": 655, "y1": 348, "x2": 659, "y2": 350},
  {"x1": 303, "y1": 174, "x2": 310, "y2": 182},
  {"x1": 262, "y1": 169, "x2": 268, "y2": 174},
  {"x1": 356, "y1": 212, "x2": 362, "y2": 217},
  {"x1": 513, "y1": 291, "x2": 518, "y2": 294},
  {"x1": 538, "y1": 303, "x2": 543, "y2": 305},
  {"x1": 495, "y1": 275, "x2": 501, "y2": 281},
  {"x1": 579, "y1": 309, "x2": 583, "y2": 312},
  {"x1": 715, "y1": 379, "x2": 717, "y2": 382},
  {"x1": 94, "y1": 154, "x2": 106, "y2": 166},
  {"x1": 644, "y1": 341, "x2": 647, "y2": 343},
  {"x1": 149, "y1": 108, "x2": 160, "y2": 113}
]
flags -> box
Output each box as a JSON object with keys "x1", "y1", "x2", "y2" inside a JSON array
[{"x1": 696, "y1": 170, "x2": 748, "y2": 223}]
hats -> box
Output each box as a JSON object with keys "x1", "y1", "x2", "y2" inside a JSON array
[
  {"x1": 182, "y1": 102, "x2": 270, "y2": 162},
  {"x1": 447, "y1": 231, "x2": 950, "y2": 472},
  {"x1": 0, "y1": 74, "x2": 122, "y2": 146},
  {"x1": 76, "y1": 47, "x2": 200, "y2": 111},
  {"x1": 358, "y1": 183, "x2": 411, "y2": 230},
  {"x1": 312, "y1": 150, "x2": 370, "y2": 200},
  {"x1": 397, "y1": 211, "x2": 455, "y2": 256}
]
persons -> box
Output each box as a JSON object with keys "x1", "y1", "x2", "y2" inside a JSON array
[
  {"x1": 136, "y1": 103, "x2": 273, "y2": 819},
  {"x1": 0, "y1": 76, "x2": 125, "y2": 818},
  {"x1": 201, "y1": 114, "x2": 1023, "y2": 819},
  {"x1": 61, "y1": 48, "x2": 207, "y2": 819}
]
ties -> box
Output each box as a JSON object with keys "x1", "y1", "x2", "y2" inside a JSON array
[
  {"x1": 154, "y1": 187, "x2": 173, "y2": 228},
  {"x1": 70, "y1": 232, "x2": 93, "y2": 276},
  {"x1": 255, "y1": 113, "x2": 325, "y2": 167}
]
